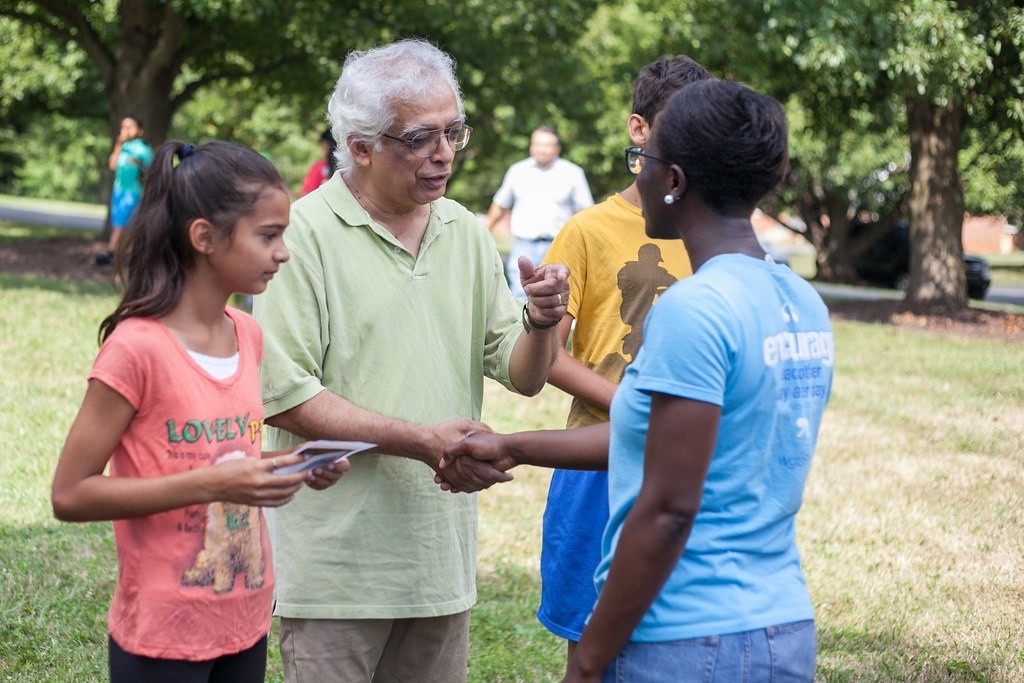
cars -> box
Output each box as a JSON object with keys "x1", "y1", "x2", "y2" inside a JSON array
[{"x1": 815, "y1": 216, "x2": 990, "y2": 297}]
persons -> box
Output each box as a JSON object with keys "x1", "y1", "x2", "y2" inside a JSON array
[
  {"x1": 56, "y1": 140, "x2": 352, "y2": 683},
  {"x1": 530, "y1": 55, "x2": 725, "y2": 677},
  {"x1": 250, "y1": 39, "x2": 571, "y2": 683},
  {"x1": 484, "y1": 127, "x2": 596, "y2": 315},
  {"x1": 104, "y1": 116, "x2": 154, "y2": 252},
  {"x1": 559, "y1": 75, "x2": 837, "y2": 683},
  {"x1": 299, "y1": 129, "x2": 333, "y2": 194}
]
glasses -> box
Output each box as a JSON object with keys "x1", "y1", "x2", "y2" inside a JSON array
[
  {"x1": 626, "y1": 146, "x2": 675, "y2": 174},
  {"x1": 376, "y1": 123, "x2": 471, "y2": 157}
]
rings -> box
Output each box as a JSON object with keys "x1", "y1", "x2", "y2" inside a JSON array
[
  {"x1": 558, "y1": 294, "x2": 562, "y2": 305},
  {"x1": 271, "y1": 457, "x2": 277, "y2": 468}
]
